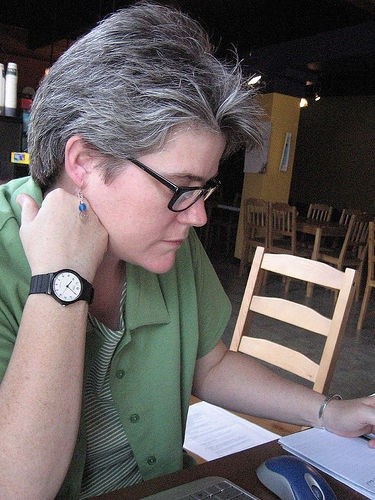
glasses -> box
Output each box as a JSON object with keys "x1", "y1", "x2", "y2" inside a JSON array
[{"x1": 127, "y1": 156, "x2": 220, "y2": 212}]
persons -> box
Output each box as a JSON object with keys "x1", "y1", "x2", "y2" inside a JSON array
[{"x1": 0, "y1": 0, "x2": 375, "y2": 500}]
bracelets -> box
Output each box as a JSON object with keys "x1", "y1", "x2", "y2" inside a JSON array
[{"x1": 318, "y1": 392, "x2": 343, "y2": 433}]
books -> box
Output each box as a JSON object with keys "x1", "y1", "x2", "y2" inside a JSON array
[{"x1": 278, "y1": 426, "x2": 375, "y2": 500}]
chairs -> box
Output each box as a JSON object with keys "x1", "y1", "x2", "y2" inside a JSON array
[
  {"x1": 199, "y1": 190, "x2": 243, "y2": 254},
  {"x1": 186, "y1": 246, "x2": 359, "y2": 464},
  {"x1": 239, "y1": 197, "x2": 375, "y2": 330}
]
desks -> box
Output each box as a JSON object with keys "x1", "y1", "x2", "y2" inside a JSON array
[
  {"x1": 276, "y1": 214, "x2": 350, "y2": 298},
  {"x1": 82, "y1": 426, "x2": 375, "y2": 500}
]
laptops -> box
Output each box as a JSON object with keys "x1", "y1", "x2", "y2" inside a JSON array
[{"x1": 136, "y1": 475, "x2": 261, "y2": 500}]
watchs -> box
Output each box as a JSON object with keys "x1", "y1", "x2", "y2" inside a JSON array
[{"x1": 28, "y1": 269, "x2": 95, "y2": 307}]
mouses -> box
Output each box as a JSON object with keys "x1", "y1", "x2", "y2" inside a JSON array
[{"x1": 255, "y1": 455, "x2": 340, "y2": 500}]
